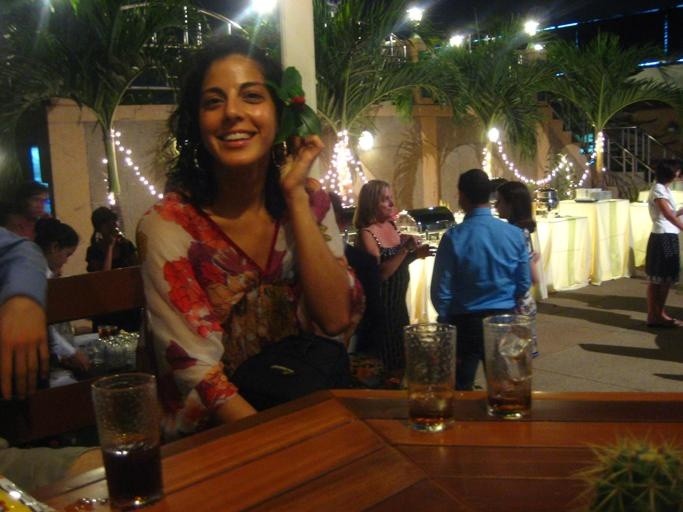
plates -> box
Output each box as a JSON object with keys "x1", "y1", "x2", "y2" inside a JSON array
[{"x1": 574, "y1": 187, "x2": 612, "y2": 201}]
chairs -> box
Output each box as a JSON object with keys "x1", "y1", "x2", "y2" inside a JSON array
[{"x1": 1, "y1": 262, "x2": 143, "y2": 451}]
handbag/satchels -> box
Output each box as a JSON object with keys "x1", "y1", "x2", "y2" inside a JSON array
[{"x1": 227, "y1": 333, "x2": 354, "y2": 411}]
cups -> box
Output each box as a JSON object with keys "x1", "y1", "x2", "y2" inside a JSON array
[
  {"x1": 88, "y1": 372, "x2": 163, "y2": 508},
  {"x1": 401, "y1": 321, "x2": 457, "y2": 435},
  {"x1": 92, "y1": 331, "x2": 140, "y2": 369},
  {"x1": 479, "y1": 312, "x2": 536, "y2": 420}
]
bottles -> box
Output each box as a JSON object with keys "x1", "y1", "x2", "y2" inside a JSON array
[{"x1": 344, "y1": 230, "x2": 349, "y2": 243}]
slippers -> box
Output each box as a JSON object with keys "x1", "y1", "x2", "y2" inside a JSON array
[{"x1": 646, "y1": 319, "x2": 680, "y2": 329}]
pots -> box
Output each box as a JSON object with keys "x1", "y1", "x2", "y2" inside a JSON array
[{"x1": 536, "y1": 187, "x2": 558, "y2": 210}]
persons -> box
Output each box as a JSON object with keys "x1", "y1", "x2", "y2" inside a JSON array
[
  {"x1": 30, "y1": 214, "x2": 80, "y2": 279},
  {"x1": 3, "y1": 178, "x2": 47, "y2": 240},
  {"x1": 134, "y1": 33, "x2": 368, "y2": 445},
  {"x1": 0, "y1": 222, "x2": 52, "y2": 406},
  {"x1": 485, "y1": 173, "x2": 508, "y2": 199},
  {"x1": 642, "y1": 159, "x2": 681, "y2": 330},
  {"x1": 493, "y1": 178, "x2": 540, "y2": 362},
  {"x1": 430, "y1": 167, "x2": 532, "y2": 391},
  {"x1": 83, "y1": 202, "x2": 137, "y2": 276},
  {"x1": 342, "y1": 178, "x2": 435, "y2": 388}
]
